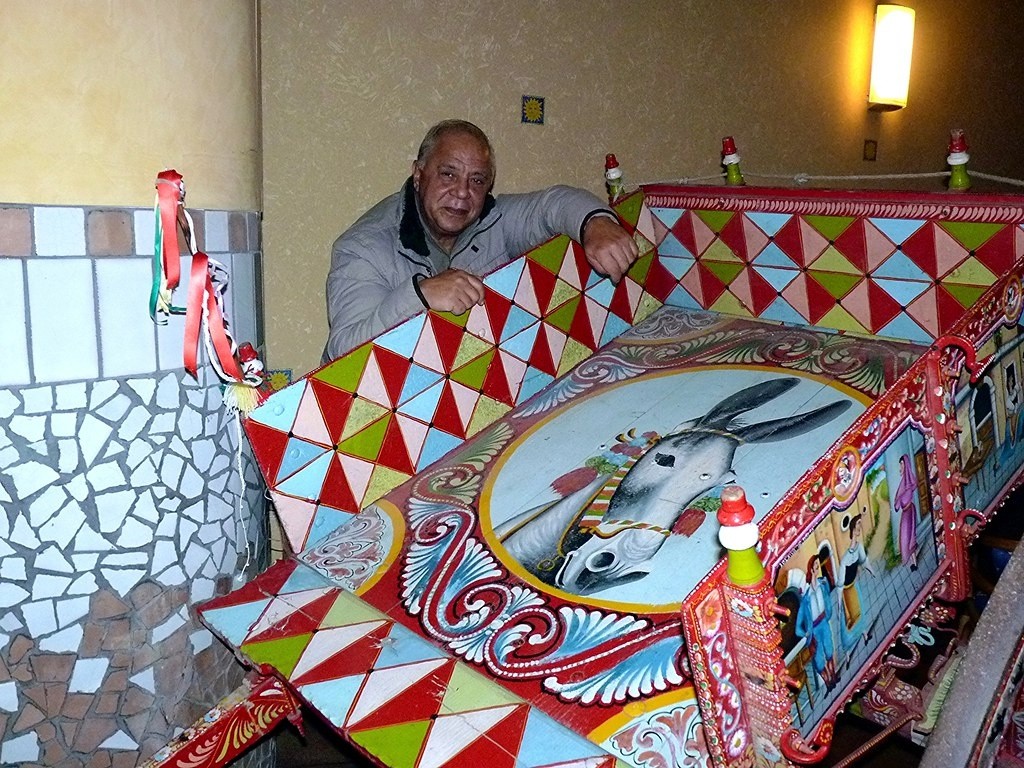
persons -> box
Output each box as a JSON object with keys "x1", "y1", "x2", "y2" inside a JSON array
[{"x1": 319, "y1": 119, "x2": 640, "y2": 368}]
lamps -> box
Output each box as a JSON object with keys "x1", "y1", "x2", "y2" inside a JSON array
[{"x1": 869, "y1": 4, "x2": 916, "y2": 113}]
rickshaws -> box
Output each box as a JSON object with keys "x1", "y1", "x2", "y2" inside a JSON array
[{"x1": 138, "y1": 183, "x2": 1023, "y2": 768}]
